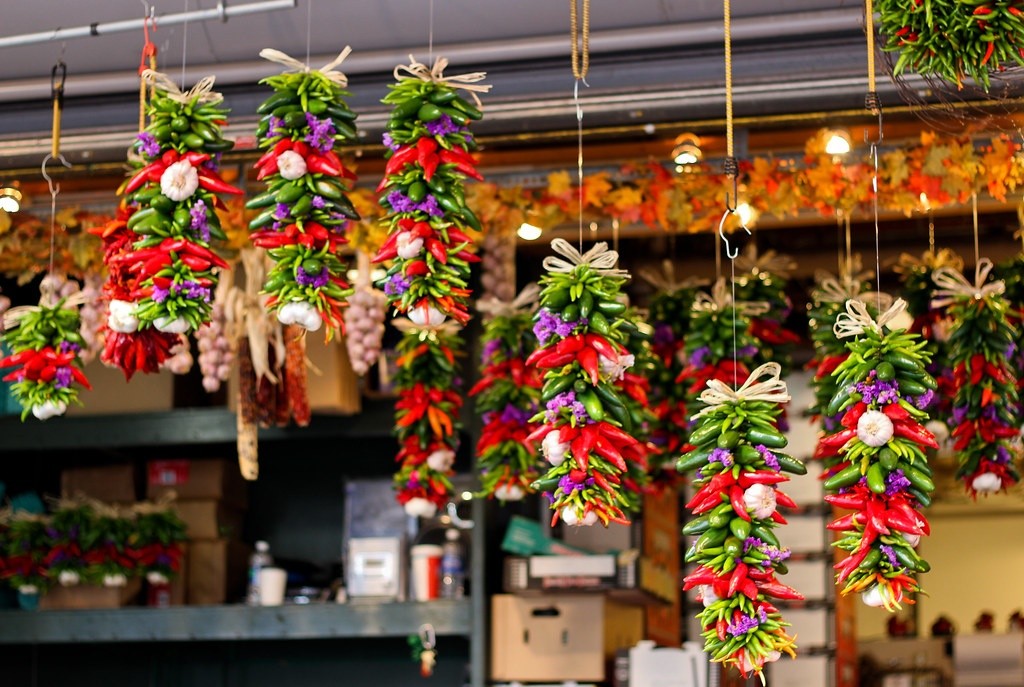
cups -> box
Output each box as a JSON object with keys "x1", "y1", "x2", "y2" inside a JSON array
[
  {"x1": 411, "y1": 545, "x2": 444, "y2": 603},
  {"x1": 257, "y1": 569, "x2": 287, "y2": 606}
]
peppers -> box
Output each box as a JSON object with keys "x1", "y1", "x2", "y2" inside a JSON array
[{"x1": 0, "y1": 0, "x2": 1024, "y2": 687}]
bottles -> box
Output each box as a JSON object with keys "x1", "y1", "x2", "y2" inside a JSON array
[
  {"x1": 439, "y1": 541, "x2": 461, "y2": 601},
  {"x1": 247, "y1": 542, "x2": 270, "y2": 606}
]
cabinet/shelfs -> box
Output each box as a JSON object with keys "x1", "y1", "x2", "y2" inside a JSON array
[{"x1": 0, "y1": 396, "x2": 487, "y2": 687}]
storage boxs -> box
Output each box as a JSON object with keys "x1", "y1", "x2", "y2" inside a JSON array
[
  {"x1": 187, "y1": 537, "x2": 251, "y2": 604},
  {"x1": 40, "y1": 584, "x2": 123, "y2": 610},
  {"x1": 154, "y1": 499, "x2": 243, "y2": 539},
  {"x1": 61, "y1": 464, "x2": 141, "y2": 503},
  {"x1": 491, "y1": 592, "x2": 643, "y2": 682},
  {"x1": 146, "y1": 459, "x2": 248, "y2": 500},
  {"x1": 64, "y1": 359, "x2": 173, "y2": 416},
  {"x1": 304, "y1": 320, "x2": 360, "y2": 416}
]
